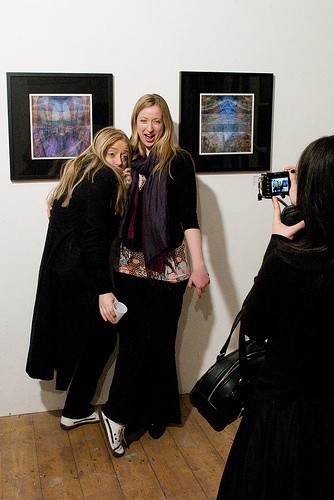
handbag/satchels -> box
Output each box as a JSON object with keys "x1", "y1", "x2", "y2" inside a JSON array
[{"x1": 189, "y1": 306, "x2": 271, "y2": 432}]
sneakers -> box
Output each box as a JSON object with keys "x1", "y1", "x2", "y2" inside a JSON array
[
  {"x1": 101, "y1": 406, "x2": 129, "y2": 457},
  {"x1": 60, "y1": 407, "x2": 100, "y2": 430}
]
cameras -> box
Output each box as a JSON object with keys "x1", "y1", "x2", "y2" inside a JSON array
[{"x1": 261, "y1": 170, "x2": 291, "y2": 198}]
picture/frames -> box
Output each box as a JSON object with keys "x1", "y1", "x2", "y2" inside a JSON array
[
  {"x1": 178, "y1": 71, "x2": 276, "y2": 173},
  {"x1": 6, "y1": 72, "x2": 114, "y2": 181}
]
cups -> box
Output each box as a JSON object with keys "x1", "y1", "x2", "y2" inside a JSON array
[{"x1": 112, "y1": 302, "x2": 127, "y2": 323}]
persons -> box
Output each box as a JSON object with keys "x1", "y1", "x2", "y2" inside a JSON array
[
  {"x1": 274, "y1": 180, "x2": 283, "y2": 192},
  {"x1": 216, "y1": 135, "x2": 334, "y2": 500},
  {"x1": 25, "y1": 127, "x2": 149, "y2": 458},
  {"x1": 113, "y1": 94, "x2": 210, "y2": 439}
]
